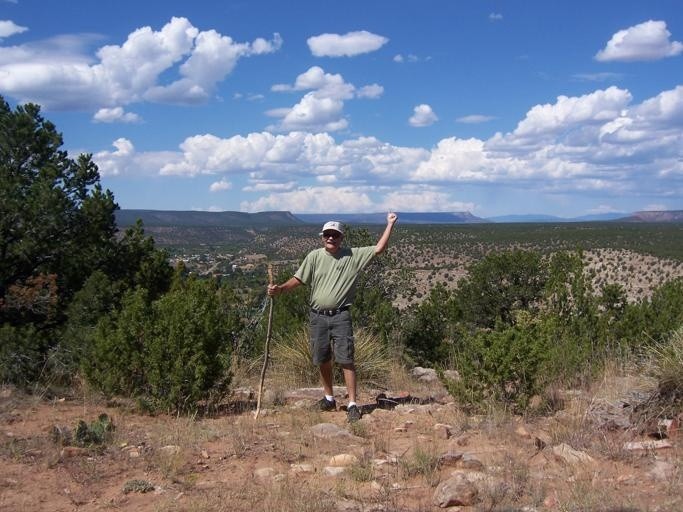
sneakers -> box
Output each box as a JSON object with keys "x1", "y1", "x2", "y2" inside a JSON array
[
  {"x1": 347, "y1": 406, "x2": 362, "y2": 421},
  {"x1": 310, "y1": 397, "x2": 337, "y2": 413}
]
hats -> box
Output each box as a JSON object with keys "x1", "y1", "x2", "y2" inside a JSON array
[{"x1": 322, "y1": 221, "x2": 346, "y2": 236}]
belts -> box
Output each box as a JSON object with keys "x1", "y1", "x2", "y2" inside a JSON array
[{"x1": 312, "y1": 308, "x2": 348, "y2": 316}]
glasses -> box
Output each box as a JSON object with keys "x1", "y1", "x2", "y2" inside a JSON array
[{"x1": 322, "y1": 232, "x2": 340, "y2": 239}]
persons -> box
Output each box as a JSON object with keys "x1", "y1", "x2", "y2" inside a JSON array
[{"x1": 267, "y1": 212, "x2": 398, "y2": 422}]
